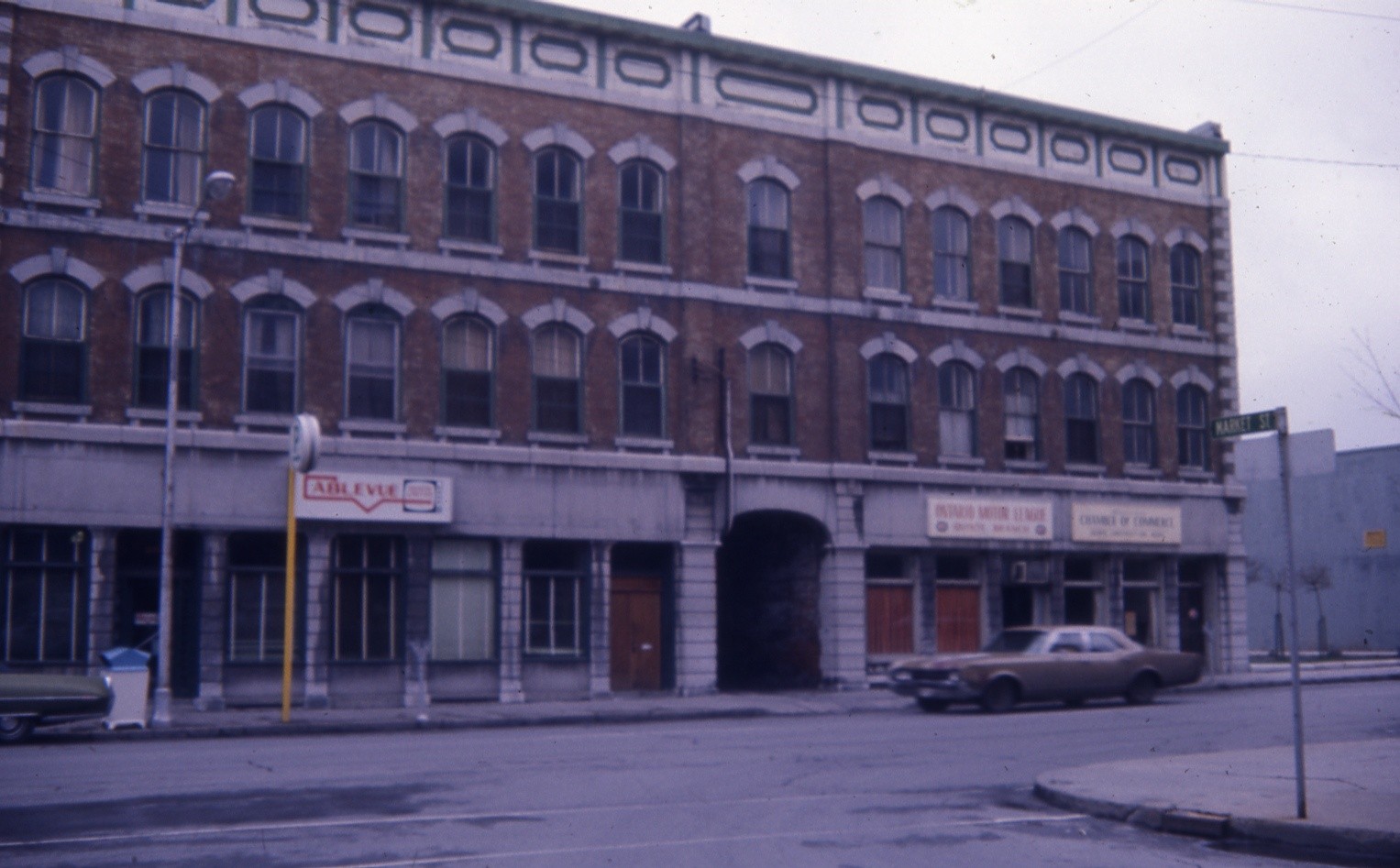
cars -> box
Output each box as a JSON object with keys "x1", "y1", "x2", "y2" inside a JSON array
[
  {"x1": 887, "y1": 624, "x2": 1204, "y2": 715},
  {"x1": 1, "y1": 671, "x2": 114, "y2": 747}
]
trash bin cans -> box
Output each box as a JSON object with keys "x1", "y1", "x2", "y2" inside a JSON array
[{"x1": 98, "y1": 648, "x2": 152, "y2": 732}]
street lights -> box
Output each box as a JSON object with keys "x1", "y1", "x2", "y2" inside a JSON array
[{"x1": 147, "y1": 171, "x2": 238, "y2": 727}]
